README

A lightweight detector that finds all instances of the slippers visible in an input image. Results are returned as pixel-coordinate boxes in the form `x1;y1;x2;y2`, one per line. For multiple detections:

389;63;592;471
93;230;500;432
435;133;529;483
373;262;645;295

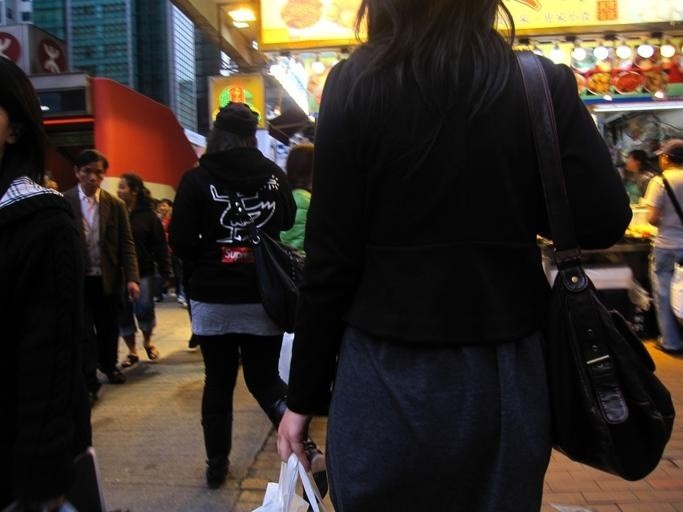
99;343;159;385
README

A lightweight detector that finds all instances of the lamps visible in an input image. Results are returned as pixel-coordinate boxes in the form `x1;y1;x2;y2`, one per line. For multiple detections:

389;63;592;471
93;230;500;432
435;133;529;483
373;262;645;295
593;43;609;60
290;55;304;73
533;45;543;55
311;54;324;73
332;55;341;65
616;40;632;60
570;43;587;62
552;43;564;62
636;40;654;59
270;56;283;75
659;37;676;58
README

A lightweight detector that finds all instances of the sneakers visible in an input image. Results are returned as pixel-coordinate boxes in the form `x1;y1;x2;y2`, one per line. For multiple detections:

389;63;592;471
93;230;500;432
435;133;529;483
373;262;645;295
187;334;200;352
204;461;228;488
301;439;327;503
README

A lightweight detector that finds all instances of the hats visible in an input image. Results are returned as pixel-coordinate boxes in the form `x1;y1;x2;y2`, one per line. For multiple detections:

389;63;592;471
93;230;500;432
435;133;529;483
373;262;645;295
654;138;683;161
215;101;258;137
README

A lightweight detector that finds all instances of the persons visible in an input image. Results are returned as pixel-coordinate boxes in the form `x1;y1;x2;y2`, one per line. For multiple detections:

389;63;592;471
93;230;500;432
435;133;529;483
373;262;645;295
643;139;683;354
168;101;328;512
60;150;141;391
0;56;105;512
118;171;198;367
621;150;654;196
277;0;632;512
280;142;314;255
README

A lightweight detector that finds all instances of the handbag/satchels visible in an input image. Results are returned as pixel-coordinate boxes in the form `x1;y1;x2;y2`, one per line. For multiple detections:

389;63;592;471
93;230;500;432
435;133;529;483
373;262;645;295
252;232;305;334
670;255;683;320
543;259;674;482
